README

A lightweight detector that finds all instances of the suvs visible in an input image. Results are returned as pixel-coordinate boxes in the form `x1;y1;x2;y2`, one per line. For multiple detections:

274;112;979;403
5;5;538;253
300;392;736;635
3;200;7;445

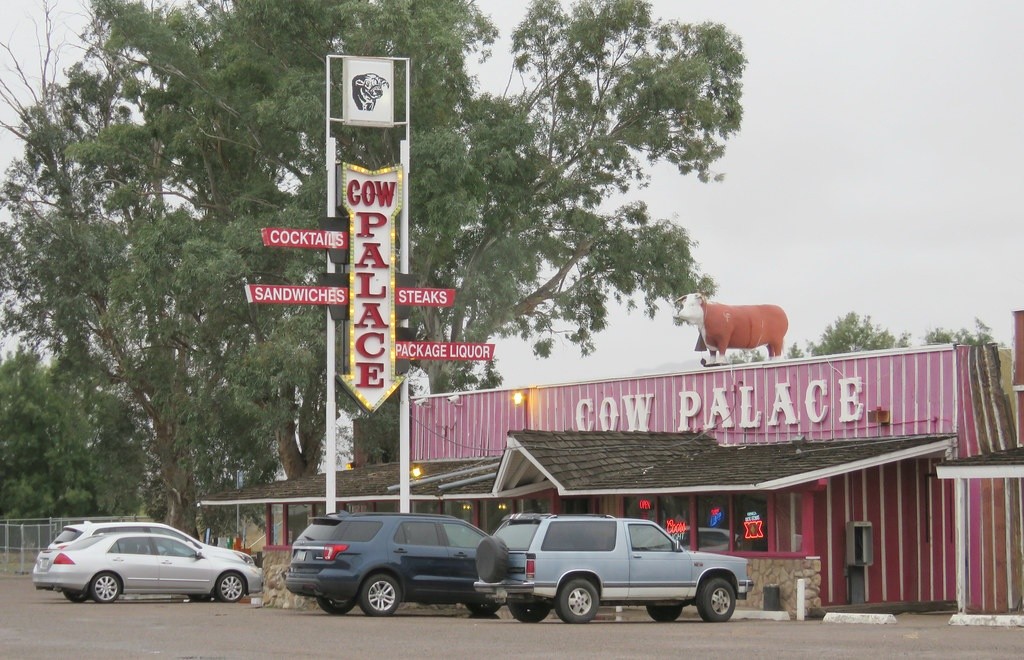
474;513;754;624
645;526;743;553
285;513;503;617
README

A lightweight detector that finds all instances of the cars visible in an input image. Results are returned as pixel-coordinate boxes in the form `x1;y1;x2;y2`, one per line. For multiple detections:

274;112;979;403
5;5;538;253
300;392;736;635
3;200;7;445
32;520;264;603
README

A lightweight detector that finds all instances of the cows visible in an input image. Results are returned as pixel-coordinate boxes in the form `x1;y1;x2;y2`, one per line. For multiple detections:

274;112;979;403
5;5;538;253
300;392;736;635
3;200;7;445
670;293;791;365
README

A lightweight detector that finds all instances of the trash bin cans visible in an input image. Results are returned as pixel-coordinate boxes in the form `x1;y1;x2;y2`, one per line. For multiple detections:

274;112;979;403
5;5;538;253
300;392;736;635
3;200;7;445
763;584;780;611
